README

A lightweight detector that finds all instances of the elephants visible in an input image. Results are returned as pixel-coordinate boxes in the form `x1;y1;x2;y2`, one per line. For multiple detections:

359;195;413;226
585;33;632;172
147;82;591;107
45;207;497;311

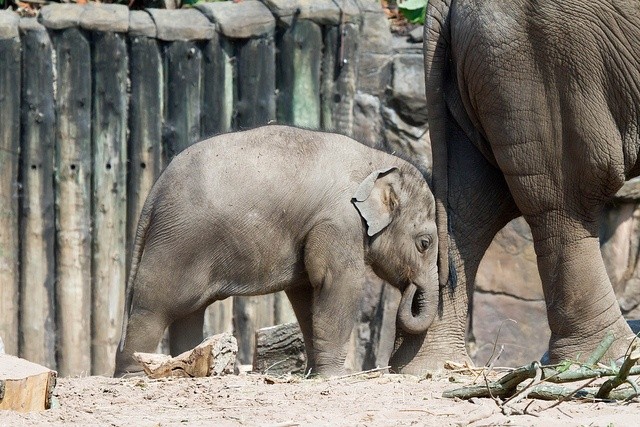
114;122;438;378
387;1;640;374
